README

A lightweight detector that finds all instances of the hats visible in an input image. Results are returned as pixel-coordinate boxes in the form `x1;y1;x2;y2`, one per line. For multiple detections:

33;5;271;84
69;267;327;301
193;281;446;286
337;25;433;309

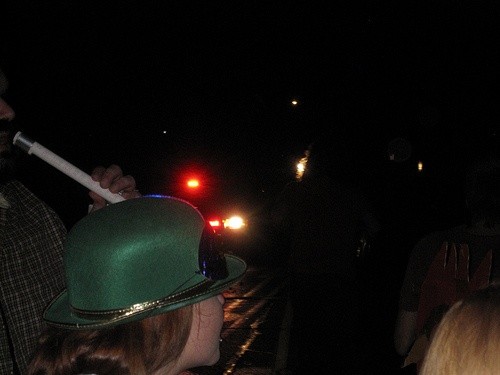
41;195;247;331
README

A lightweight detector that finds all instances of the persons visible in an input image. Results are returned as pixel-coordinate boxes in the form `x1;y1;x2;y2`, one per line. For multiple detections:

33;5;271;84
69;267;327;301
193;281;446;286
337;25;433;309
390;151;500;371
26;194;248;375
1;74;144;374
269;134;382;370
